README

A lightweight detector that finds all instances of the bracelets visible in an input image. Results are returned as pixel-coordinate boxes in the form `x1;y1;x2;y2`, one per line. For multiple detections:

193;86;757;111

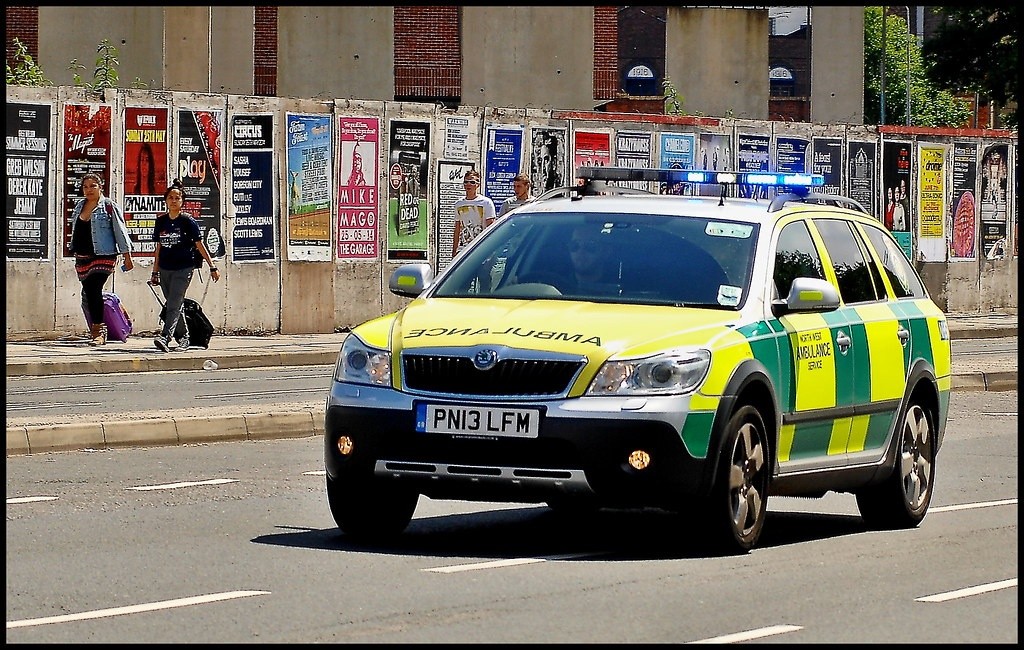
151;272;158;278
211;267;218;272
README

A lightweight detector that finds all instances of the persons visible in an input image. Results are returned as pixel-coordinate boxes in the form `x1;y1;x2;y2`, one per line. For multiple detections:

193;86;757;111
508;223;617;290
133;144;157;196
498;172;536;216
539;136;563;198
407;166;420;196
149;187;221;353
900;179;910;232
347;141;367;186
452;170;495;294
70;174;134;346
892;186;906;231
884;187;895;232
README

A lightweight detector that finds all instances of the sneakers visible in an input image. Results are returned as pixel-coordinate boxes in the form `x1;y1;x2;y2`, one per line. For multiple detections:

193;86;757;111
153;338;171;353
175;339;190;352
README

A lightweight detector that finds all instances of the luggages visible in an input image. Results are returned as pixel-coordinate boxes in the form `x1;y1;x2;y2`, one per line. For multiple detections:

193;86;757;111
146;280;215;350
80;290;133;344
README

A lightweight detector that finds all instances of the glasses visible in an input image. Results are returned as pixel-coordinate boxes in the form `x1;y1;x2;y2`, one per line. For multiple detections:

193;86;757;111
568;240;600;253
464;180;480;186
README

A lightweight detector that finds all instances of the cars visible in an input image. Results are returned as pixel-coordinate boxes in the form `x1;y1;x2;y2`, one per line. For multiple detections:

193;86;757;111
323;168;954;551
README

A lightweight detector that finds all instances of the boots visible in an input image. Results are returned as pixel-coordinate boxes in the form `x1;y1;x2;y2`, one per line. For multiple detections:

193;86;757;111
88;326;95;345
90;324;107;346
